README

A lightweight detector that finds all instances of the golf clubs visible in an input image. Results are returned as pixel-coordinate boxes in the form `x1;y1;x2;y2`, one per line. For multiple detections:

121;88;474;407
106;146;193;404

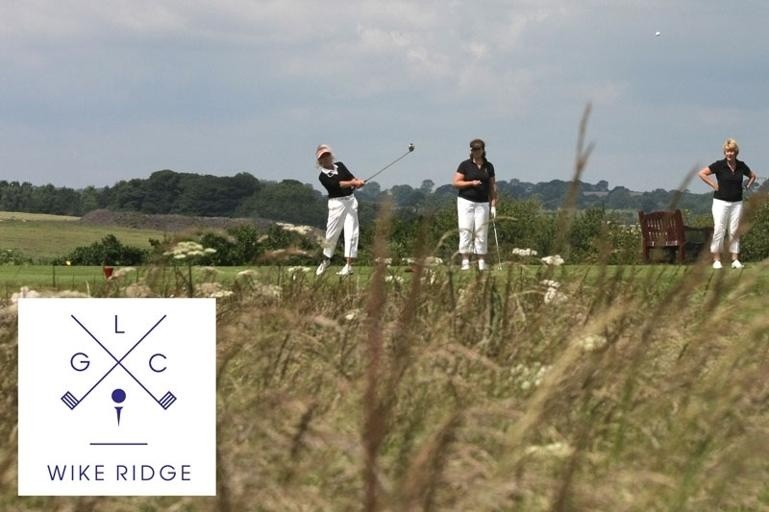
491;214;503;271
352;143;415;189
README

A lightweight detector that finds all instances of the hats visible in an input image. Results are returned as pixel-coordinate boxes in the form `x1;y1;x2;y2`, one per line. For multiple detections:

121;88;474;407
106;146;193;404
469;138;486;148
316;144;331;160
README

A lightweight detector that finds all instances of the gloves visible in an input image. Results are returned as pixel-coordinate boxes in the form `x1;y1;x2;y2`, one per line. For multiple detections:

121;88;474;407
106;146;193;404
490;206;497;219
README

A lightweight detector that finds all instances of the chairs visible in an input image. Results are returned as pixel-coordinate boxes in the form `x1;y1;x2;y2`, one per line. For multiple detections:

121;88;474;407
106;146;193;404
638;208;715;264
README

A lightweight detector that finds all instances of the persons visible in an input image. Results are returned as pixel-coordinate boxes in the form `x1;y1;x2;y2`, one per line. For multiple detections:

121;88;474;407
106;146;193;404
315;144;367;277
451;139;497;271
697;138;756;270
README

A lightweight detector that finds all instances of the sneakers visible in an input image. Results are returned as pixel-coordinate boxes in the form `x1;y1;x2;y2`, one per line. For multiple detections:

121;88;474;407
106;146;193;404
336;263;354;275
712;259;746;270
315;258;334;275
460;259;489;271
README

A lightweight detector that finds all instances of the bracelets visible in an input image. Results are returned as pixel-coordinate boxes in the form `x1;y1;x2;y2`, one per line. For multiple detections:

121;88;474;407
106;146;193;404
744;184;750;191
